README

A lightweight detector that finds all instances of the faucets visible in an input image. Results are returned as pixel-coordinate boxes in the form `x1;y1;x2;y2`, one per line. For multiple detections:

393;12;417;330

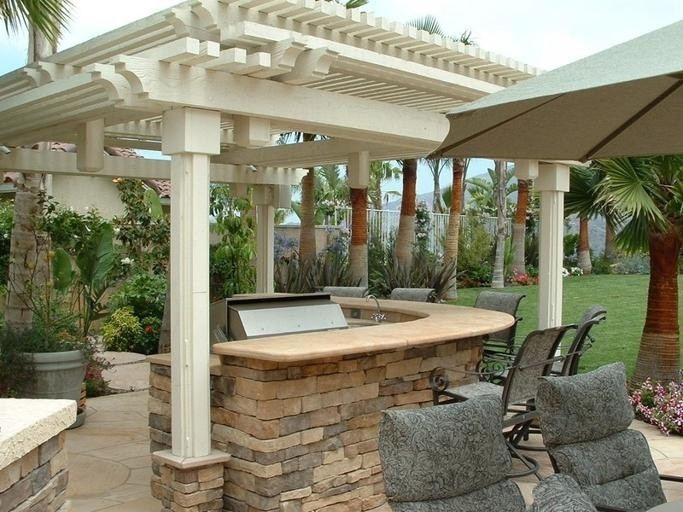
365;294;386;323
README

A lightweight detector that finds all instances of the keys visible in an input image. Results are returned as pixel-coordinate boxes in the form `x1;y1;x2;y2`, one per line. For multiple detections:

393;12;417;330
424;16;683;164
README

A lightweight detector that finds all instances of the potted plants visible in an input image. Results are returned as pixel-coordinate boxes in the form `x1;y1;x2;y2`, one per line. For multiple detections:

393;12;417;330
9;190;85;407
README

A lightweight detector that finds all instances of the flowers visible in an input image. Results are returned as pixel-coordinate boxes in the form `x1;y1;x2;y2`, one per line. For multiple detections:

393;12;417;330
629;376;683;437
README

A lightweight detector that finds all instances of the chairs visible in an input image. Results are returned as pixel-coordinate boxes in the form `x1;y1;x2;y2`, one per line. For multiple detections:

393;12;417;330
389;287;436;303
473;290;527;360
428;303;608;479
378;394;529;511
529;361;681;511
323;285;369;297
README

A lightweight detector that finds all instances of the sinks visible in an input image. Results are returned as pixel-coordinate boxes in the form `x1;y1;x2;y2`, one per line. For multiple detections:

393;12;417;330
339;321;380;329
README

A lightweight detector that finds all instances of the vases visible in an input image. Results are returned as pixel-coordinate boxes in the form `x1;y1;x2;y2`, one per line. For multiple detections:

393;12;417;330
67;380;86;428
